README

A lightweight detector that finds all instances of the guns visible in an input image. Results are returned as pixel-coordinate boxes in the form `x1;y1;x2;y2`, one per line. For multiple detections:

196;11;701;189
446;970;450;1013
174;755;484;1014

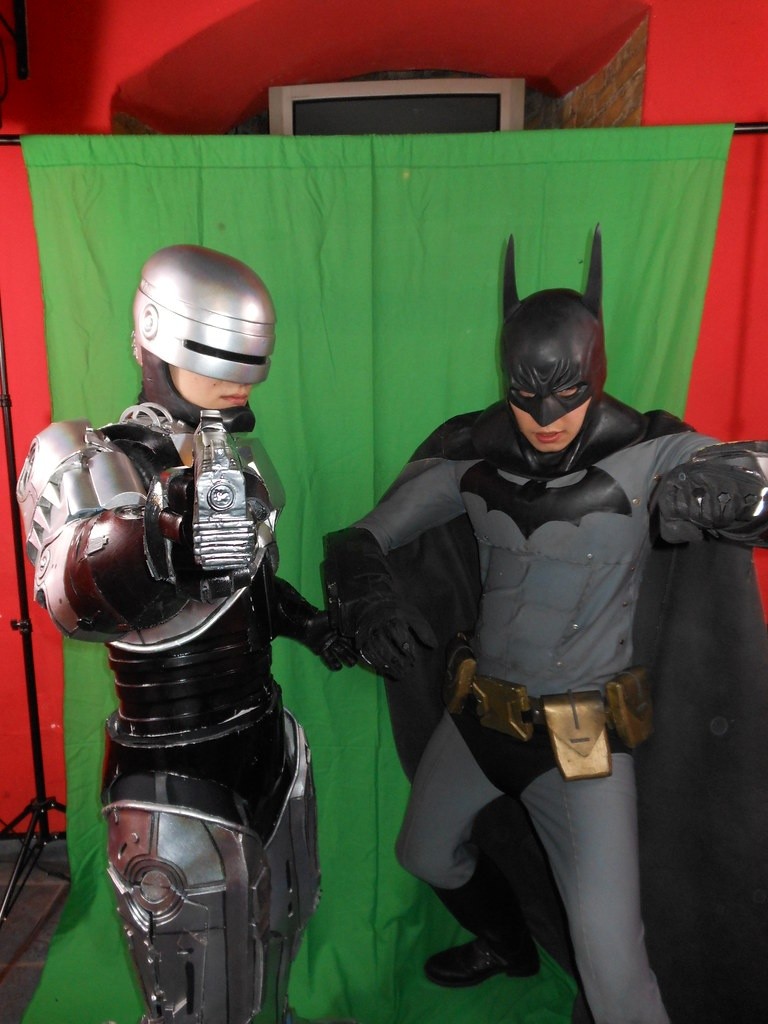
187;408;257;571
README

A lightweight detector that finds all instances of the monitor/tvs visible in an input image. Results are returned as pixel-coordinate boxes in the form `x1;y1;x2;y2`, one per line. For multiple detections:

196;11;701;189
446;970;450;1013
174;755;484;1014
270;79;528;135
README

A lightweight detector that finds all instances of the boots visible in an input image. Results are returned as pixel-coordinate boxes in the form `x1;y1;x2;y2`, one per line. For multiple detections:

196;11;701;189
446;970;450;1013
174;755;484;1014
424;846;543;987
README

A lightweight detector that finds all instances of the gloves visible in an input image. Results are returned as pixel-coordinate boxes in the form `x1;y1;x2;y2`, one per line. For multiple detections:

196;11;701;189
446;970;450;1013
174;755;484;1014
654;462;768;543
322;551;438;679
144;466;280;599
303;612;359;671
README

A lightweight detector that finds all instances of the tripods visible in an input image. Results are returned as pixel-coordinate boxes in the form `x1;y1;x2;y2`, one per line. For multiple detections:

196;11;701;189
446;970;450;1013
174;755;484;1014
0;318;65;930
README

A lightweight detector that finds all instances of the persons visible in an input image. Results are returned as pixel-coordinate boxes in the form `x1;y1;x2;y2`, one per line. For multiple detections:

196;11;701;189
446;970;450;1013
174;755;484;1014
16;245;359;1023
318;230;768;1024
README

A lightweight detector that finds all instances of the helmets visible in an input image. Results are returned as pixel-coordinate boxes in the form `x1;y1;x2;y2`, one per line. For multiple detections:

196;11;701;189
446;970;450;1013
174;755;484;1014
132;244;277;384
